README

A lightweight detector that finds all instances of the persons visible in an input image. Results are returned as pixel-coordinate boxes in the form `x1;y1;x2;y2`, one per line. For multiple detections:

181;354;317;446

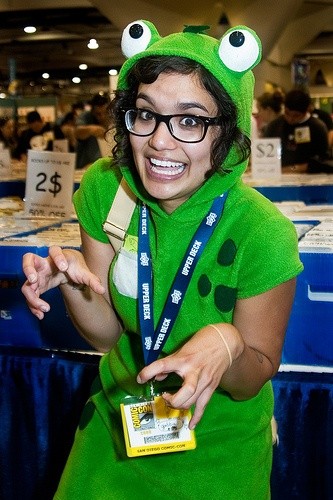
1;86;332;172
20;15;303;500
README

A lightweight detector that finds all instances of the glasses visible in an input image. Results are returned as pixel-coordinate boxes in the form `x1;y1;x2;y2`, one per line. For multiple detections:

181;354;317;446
123;108;222;143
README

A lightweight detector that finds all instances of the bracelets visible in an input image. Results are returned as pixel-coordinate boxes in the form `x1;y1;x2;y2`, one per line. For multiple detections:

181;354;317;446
204;322;234;370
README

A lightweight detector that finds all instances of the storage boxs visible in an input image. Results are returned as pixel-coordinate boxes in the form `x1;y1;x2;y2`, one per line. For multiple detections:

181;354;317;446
0;176;95;352
252;185;333;369
